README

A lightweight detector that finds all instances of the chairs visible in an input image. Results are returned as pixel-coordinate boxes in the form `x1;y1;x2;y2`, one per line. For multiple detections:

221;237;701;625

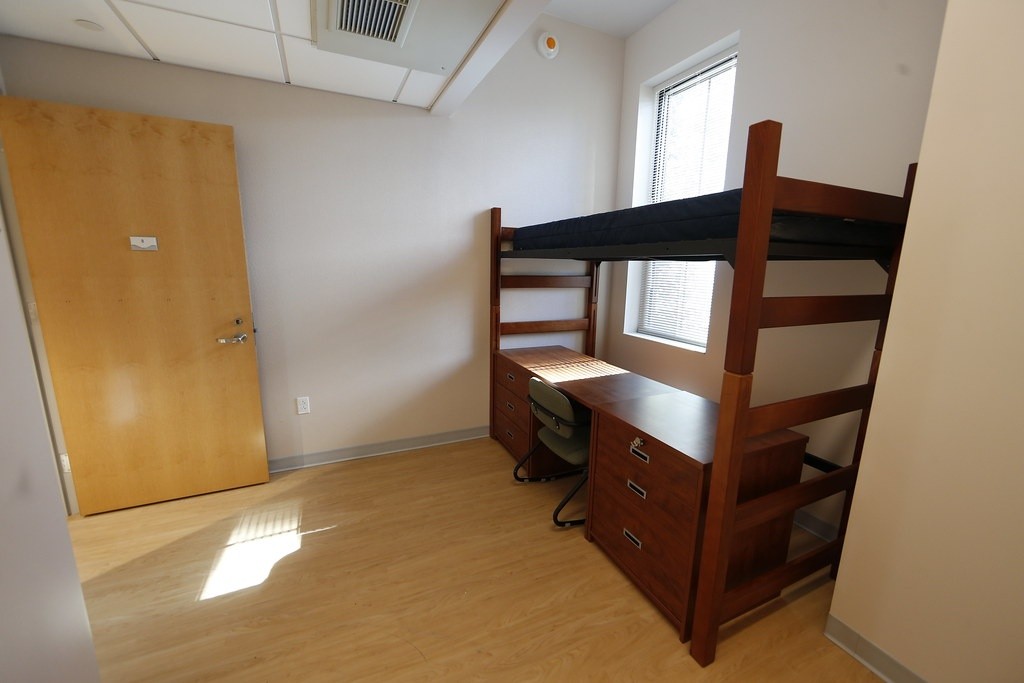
513;377;588;527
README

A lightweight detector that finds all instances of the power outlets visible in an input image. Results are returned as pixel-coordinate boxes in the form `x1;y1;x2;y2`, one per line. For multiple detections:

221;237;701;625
297;396;310;415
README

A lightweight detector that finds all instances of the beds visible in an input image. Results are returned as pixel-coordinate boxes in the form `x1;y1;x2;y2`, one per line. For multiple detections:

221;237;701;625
489;122;917;668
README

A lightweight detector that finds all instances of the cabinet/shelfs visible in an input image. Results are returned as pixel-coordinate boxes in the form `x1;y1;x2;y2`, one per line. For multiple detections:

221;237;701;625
491;346;809;645
